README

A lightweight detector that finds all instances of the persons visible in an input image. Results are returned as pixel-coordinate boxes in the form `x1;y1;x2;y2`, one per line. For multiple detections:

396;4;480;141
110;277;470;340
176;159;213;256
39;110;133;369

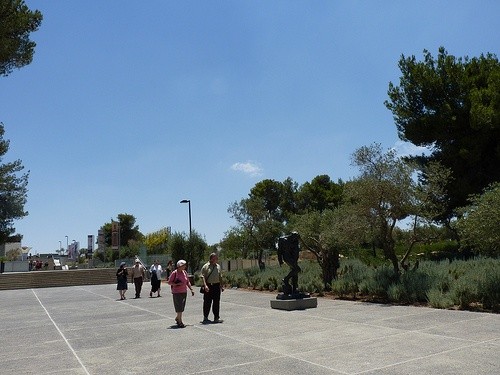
30;256;49;270
278;230;303;293
149;259;166;297
167;260;194;328
200;253;225;324
166;261;172;280
131;259;145;299
116;262;129;300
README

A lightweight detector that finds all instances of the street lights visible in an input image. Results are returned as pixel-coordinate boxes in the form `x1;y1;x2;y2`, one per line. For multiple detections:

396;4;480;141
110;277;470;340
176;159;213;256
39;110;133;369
179;200;192;243
58;240;62;250
64;235;68;246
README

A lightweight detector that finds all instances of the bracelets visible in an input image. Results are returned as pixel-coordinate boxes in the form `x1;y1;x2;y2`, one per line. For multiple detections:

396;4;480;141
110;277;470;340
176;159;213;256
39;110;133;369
190;288;193;290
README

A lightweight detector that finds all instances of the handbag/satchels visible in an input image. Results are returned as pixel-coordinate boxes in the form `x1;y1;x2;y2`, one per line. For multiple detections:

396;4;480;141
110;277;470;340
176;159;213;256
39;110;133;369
200;285;206;293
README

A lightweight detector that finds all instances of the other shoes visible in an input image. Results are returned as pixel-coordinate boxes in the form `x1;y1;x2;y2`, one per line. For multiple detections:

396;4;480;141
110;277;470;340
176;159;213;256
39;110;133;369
175;318;185;328
214;319;223;323
203;319;214;323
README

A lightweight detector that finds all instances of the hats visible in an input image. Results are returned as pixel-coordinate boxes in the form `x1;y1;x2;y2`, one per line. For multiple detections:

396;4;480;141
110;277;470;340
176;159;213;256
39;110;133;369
135;259;140;263
177;260;187;267
